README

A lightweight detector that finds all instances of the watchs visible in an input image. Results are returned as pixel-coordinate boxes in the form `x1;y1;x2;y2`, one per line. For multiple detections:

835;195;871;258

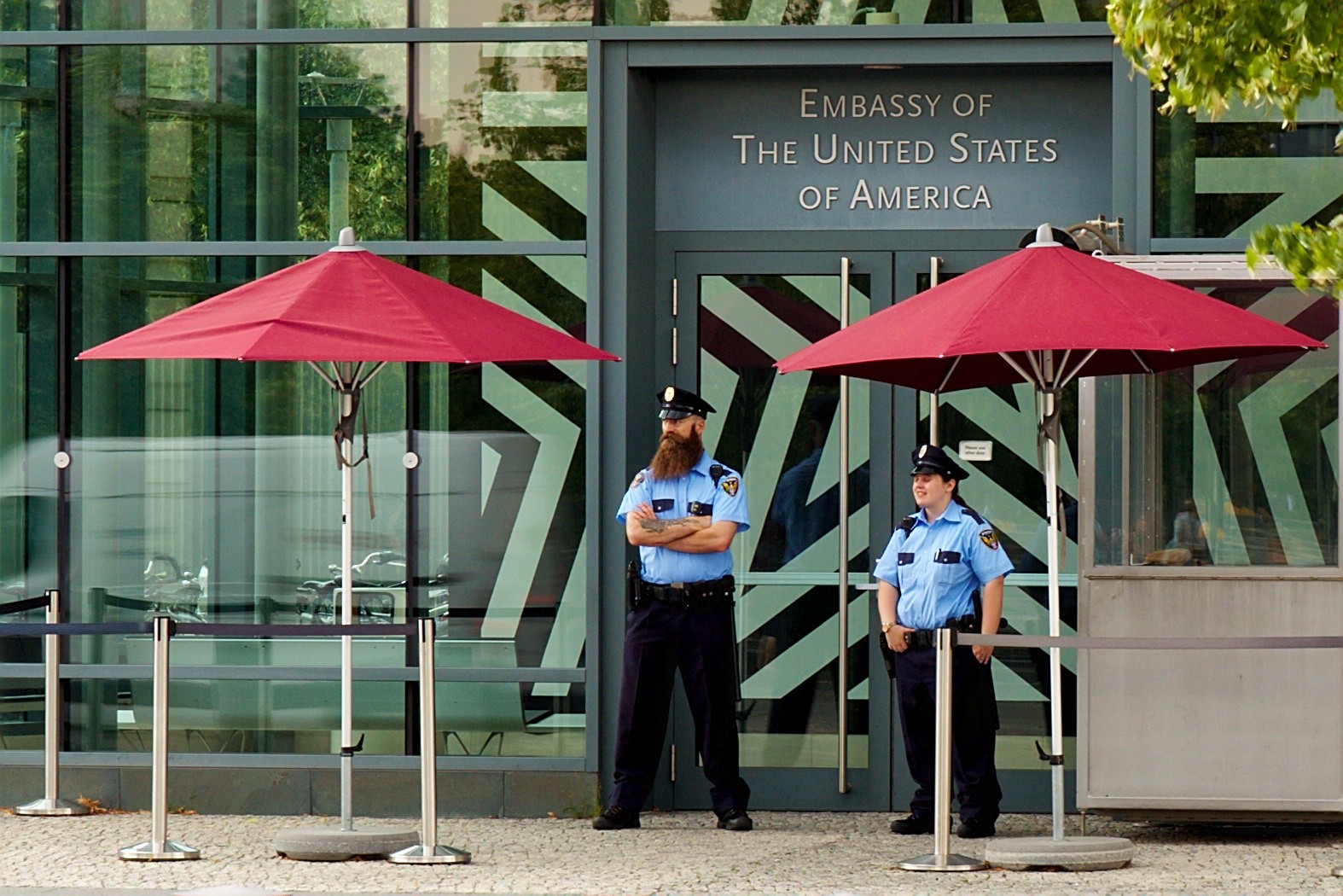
882;622;897;633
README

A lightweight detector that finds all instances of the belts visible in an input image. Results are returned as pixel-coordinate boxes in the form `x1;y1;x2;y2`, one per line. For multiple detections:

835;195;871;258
903;621;982;648
640;582;733;606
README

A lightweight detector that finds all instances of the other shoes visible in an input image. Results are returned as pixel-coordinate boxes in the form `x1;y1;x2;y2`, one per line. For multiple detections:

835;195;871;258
890;813;954;835
957;821;996;839
717;812;753;831
592;811;641;831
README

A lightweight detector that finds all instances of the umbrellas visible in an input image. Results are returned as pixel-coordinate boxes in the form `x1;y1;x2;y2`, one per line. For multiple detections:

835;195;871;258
72;224;623;863
771;221;1330;873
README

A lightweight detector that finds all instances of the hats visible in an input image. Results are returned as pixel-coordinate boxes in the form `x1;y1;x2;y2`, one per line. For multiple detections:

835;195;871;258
909;444;970;482
656;385;716;420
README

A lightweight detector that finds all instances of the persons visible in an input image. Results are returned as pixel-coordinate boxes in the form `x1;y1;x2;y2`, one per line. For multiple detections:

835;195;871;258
872;445;1016;838
590;385;755;833
